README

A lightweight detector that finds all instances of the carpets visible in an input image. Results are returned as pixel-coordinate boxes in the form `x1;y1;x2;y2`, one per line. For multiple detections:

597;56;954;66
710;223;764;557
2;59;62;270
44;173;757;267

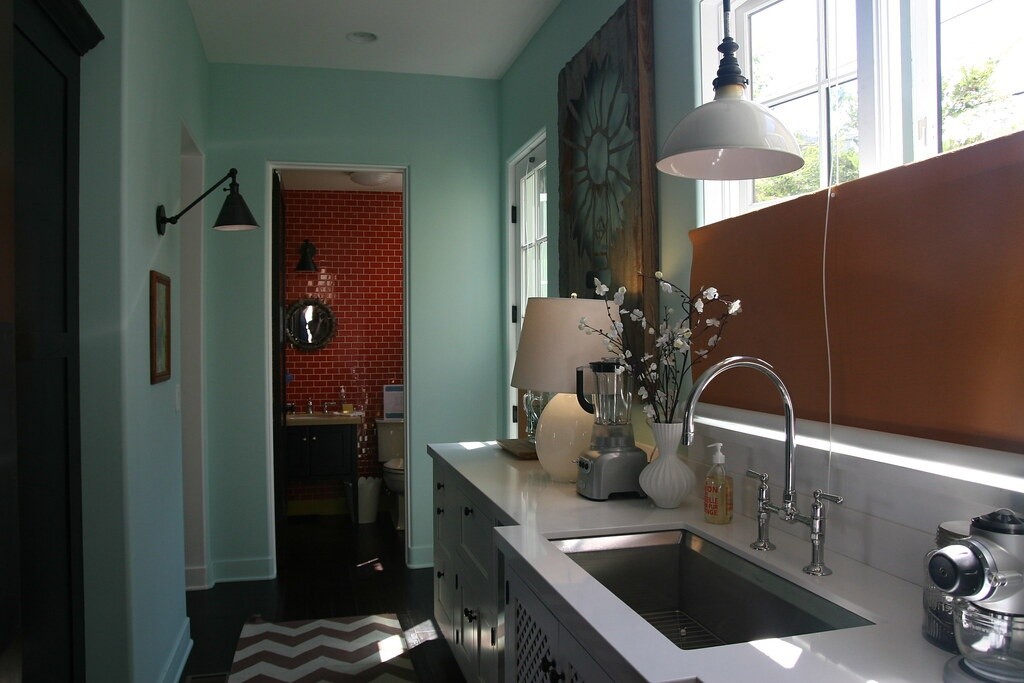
226;613;420;683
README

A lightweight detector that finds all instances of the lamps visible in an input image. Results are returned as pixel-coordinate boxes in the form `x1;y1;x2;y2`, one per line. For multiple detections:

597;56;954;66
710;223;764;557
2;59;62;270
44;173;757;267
295;240;318;271
351;171;392;186
511;298;625;484
655;0;805;180
155;168;262;235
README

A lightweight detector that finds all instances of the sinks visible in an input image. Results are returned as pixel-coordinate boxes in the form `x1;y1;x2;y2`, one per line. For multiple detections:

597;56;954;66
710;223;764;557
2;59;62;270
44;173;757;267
549;528;877;651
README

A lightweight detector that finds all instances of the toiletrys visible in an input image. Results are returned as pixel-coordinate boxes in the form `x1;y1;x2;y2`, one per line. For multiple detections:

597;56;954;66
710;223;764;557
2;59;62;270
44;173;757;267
338;385;347;412
704;442;734;525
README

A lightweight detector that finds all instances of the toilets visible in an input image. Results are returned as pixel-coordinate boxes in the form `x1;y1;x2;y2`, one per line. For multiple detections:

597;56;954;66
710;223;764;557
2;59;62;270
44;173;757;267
375;419;405;530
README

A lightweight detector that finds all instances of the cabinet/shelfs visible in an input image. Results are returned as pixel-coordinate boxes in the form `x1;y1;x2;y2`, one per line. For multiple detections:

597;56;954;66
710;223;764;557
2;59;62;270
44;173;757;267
286;425;356;477
427;442;498;683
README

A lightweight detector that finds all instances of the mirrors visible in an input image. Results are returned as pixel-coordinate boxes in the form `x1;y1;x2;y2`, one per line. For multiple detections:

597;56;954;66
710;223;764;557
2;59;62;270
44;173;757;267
284;299;335;348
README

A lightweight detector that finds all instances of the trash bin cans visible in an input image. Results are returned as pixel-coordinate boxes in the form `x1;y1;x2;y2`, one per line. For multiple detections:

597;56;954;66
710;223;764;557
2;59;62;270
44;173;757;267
345;459;384;529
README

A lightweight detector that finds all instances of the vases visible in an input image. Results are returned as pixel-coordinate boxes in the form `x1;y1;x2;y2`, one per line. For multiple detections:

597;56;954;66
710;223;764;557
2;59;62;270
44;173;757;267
639;421;702;508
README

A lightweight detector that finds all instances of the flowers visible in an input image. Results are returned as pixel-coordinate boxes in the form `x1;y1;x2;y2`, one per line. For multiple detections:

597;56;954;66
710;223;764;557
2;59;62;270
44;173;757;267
579;271;745;424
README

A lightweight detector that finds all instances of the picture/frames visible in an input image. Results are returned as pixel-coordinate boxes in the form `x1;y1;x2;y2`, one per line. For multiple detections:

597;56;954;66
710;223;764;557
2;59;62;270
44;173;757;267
149;270;173;385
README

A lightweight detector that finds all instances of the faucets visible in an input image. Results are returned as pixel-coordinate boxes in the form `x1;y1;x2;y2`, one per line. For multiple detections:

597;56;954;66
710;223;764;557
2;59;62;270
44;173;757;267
306;399;314;414
681;355;845;577
